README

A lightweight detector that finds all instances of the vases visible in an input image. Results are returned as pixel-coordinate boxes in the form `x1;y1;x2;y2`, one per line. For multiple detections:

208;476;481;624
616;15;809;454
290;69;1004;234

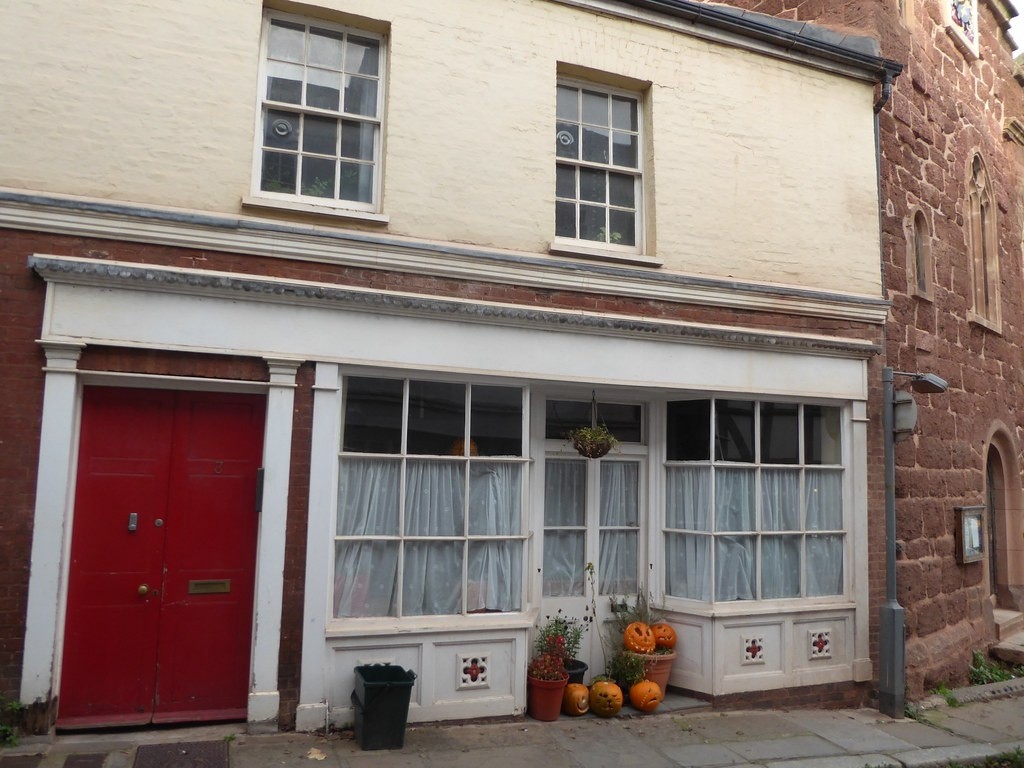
352;664;417;752
526;673;569;721
563;656;588;684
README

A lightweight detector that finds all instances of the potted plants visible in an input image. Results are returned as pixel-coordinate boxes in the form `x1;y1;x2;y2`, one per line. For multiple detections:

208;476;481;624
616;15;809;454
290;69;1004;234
568;426;624;459
598;583;679;702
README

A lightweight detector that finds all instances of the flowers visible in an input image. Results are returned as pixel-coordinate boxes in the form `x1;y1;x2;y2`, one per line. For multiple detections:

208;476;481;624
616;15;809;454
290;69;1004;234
527;605;593;680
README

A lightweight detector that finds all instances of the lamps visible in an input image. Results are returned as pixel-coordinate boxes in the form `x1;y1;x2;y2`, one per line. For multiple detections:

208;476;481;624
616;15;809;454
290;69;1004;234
894;371;949;393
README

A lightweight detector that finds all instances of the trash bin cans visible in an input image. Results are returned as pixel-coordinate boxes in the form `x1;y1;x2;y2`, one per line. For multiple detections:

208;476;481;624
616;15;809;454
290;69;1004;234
351;664;418;751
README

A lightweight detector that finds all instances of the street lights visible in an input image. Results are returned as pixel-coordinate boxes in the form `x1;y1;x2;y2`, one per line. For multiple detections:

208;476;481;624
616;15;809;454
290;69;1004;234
879;365;951;720
